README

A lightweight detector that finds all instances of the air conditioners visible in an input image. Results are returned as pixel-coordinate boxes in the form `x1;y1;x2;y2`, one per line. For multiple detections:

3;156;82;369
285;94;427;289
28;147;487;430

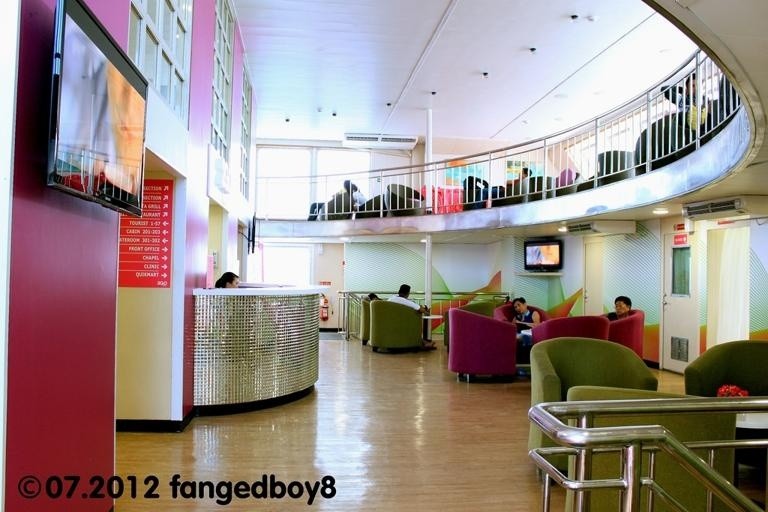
680;195;768;219
568;220;637;237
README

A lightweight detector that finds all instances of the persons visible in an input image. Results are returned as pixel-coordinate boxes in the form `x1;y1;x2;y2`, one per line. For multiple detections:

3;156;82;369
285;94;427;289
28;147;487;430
510;297;541;377
385;283;437;350
61;60;146;212
604;295;632;322
658;71;712;145
462;174;488;190
469;167;532;208
213;271;240;288
306;179;357;221
368;293;379;301
532;244;560;266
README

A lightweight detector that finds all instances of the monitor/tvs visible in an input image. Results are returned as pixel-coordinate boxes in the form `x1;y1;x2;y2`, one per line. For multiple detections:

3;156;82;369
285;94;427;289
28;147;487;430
43;1;149;220
524;239;563;273
252;216;255;253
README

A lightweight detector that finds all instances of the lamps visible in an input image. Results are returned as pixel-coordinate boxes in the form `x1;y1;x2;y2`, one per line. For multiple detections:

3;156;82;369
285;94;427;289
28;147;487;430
558;220;568;233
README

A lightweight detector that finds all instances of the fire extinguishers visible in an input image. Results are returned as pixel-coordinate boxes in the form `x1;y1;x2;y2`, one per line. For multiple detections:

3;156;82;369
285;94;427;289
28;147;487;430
320;294;329;321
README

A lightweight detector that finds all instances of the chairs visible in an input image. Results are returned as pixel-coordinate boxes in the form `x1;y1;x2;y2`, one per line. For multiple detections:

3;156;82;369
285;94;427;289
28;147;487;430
357;294;768;512
316;73;742;218
